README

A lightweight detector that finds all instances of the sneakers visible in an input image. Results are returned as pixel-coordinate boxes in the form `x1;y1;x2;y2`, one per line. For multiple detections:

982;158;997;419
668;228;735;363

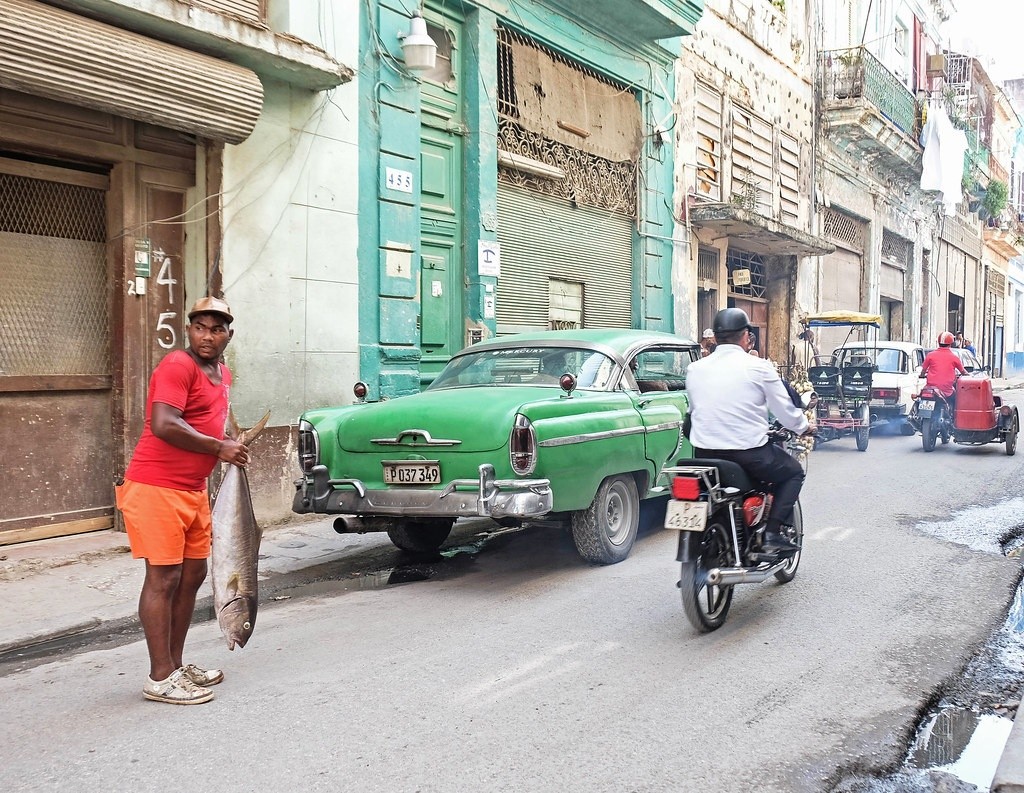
177;663;224;687
142;670;214;705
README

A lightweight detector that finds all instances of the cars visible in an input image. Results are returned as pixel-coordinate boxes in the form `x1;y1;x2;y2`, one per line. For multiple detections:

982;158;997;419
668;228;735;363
290;327;702;565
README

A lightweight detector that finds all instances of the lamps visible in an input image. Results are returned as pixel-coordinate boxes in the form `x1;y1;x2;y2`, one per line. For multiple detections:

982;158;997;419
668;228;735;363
396;9;439;70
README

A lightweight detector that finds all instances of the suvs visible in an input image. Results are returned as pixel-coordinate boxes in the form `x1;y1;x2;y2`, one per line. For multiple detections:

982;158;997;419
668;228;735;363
925;348;991;378
829;340;929;436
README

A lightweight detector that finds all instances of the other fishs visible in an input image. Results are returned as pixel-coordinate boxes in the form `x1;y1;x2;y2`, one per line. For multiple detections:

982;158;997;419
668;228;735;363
209;400;272;652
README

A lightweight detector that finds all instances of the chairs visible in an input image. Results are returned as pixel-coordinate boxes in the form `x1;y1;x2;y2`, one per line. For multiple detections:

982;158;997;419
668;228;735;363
841;355;873;398
636;381;669;393
852;354;868;365
808;354;840;395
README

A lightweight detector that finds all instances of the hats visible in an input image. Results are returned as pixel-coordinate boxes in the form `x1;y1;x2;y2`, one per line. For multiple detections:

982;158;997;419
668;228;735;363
703;328;714;338
964;337;972;344
188;296;234;325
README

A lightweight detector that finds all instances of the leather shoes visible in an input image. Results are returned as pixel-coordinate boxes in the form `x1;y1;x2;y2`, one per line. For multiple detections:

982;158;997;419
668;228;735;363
765;531;802;550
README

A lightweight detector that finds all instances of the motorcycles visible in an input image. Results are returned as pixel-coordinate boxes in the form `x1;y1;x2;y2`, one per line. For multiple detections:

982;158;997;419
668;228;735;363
797;310;885;451
658;378;818;633
907;365;1020;456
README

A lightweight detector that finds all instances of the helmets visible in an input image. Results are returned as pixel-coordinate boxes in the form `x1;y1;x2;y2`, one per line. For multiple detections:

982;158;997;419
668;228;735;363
938;331;955;344
713;308;753;332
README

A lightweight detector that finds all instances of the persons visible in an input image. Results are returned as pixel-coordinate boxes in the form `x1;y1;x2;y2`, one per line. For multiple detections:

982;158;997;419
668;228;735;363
115;297;251;707
686;305;820;553
920;330;976;423
